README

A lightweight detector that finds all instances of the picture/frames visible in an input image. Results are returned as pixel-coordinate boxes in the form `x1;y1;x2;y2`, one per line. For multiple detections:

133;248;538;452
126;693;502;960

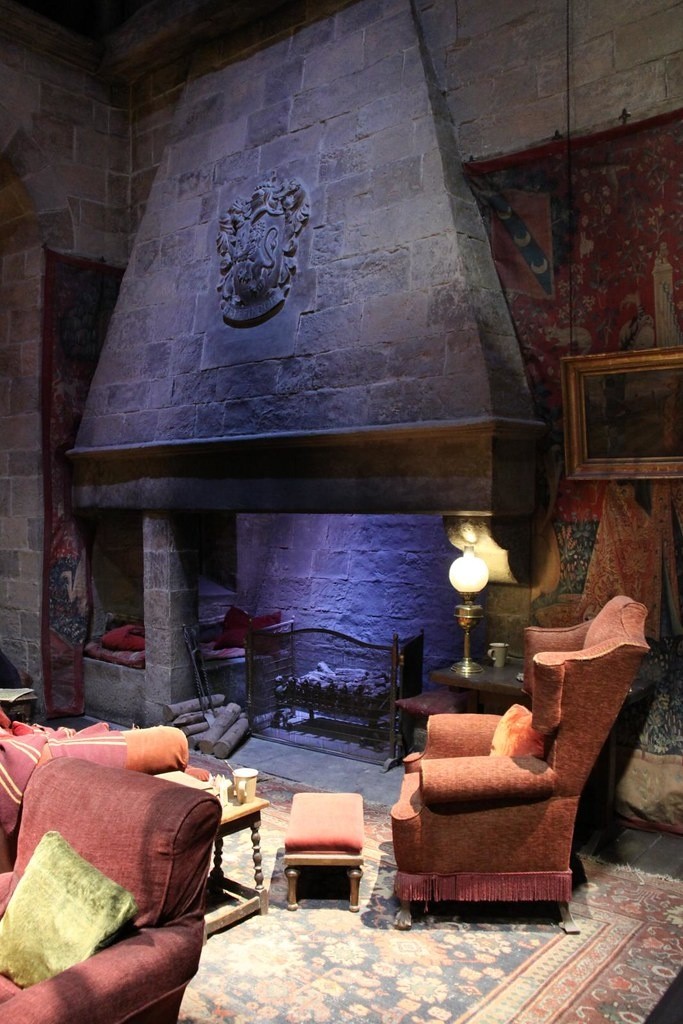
560;344;683;481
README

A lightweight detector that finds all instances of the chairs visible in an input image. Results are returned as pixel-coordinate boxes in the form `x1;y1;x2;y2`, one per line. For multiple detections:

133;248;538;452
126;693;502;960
388;595;650;934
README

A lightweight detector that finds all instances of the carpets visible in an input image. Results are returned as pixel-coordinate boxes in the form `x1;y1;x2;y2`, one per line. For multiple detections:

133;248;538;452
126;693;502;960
177;751;683;1024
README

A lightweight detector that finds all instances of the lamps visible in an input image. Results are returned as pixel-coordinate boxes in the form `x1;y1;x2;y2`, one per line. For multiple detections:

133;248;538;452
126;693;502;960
449;546;489;674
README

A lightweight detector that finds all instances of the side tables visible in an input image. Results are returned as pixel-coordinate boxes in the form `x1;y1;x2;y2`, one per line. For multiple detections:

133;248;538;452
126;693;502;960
430;664;531;716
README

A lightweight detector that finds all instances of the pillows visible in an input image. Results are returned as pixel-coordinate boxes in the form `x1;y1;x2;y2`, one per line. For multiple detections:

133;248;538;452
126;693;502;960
100;624;144;650
488;703;544;759
211;606;281;658
0;831;138;988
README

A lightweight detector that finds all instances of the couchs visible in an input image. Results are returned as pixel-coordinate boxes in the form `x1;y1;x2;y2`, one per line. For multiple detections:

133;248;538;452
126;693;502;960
0;756;223;1024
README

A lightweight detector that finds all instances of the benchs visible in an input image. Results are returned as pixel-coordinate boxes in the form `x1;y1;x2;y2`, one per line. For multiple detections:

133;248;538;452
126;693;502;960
0;705;210;874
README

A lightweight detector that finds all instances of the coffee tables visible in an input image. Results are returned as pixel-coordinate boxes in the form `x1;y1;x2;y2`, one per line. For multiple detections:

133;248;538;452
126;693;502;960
153;771;270;947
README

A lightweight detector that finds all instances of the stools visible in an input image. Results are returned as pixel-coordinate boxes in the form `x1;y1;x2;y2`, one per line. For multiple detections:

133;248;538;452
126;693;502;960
283;792;364;912
